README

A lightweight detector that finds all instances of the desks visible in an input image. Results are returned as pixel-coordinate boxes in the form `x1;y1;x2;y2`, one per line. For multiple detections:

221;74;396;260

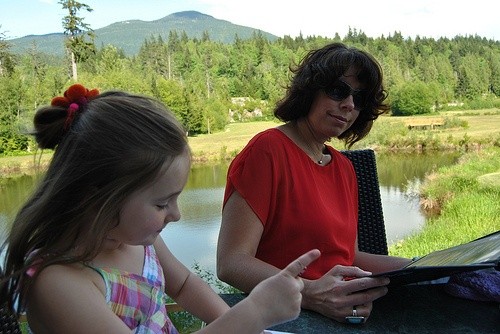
214;293;499;334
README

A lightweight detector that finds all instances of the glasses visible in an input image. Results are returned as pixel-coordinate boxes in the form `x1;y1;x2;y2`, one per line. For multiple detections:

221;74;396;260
312;80;372;110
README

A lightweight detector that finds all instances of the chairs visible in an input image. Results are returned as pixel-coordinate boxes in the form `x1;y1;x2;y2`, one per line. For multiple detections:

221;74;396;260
340;149;388;255
0;268;185;334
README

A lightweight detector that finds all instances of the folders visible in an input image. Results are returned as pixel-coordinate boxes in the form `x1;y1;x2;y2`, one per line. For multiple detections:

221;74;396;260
369;230;500;285
